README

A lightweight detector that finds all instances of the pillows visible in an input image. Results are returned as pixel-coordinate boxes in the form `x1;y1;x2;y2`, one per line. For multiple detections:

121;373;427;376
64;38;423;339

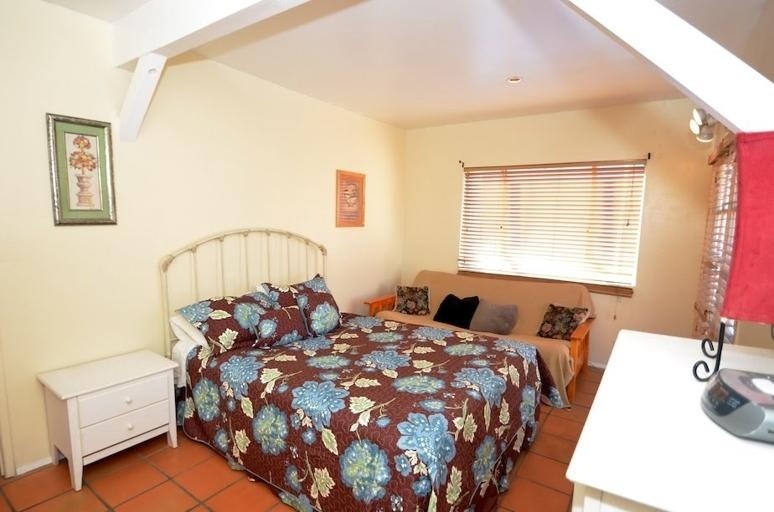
171;324;190;341
536;302;591;340
433;294;480;330
393;285;430;315
469;297;518;335
248;304;309;351
174;290;280;357
170;316;209;349
287;273;343;337
259;279;298;307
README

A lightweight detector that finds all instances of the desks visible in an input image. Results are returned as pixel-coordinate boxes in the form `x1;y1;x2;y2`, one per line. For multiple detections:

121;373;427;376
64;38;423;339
565;329;773;512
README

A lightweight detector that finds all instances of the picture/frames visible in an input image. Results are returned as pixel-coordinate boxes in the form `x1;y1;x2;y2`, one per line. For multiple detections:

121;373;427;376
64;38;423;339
47;113;117;224
336;169;365;228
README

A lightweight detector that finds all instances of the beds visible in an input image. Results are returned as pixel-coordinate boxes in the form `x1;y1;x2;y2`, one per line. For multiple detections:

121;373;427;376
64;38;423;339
158;228;570;512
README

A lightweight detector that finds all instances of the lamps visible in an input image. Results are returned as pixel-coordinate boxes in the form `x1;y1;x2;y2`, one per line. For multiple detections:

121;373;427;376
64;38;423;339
689;107;719;143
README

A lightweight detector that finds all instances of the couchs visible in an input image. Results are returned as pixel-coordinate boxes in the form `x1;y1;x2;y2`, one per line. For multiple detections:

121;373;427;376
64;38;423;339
364;269;596;405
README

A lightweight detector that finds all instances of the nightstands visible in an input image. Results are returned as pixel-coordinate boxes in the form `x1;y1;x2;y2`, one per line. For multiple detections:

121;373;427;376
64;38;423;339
36;349;179;492
